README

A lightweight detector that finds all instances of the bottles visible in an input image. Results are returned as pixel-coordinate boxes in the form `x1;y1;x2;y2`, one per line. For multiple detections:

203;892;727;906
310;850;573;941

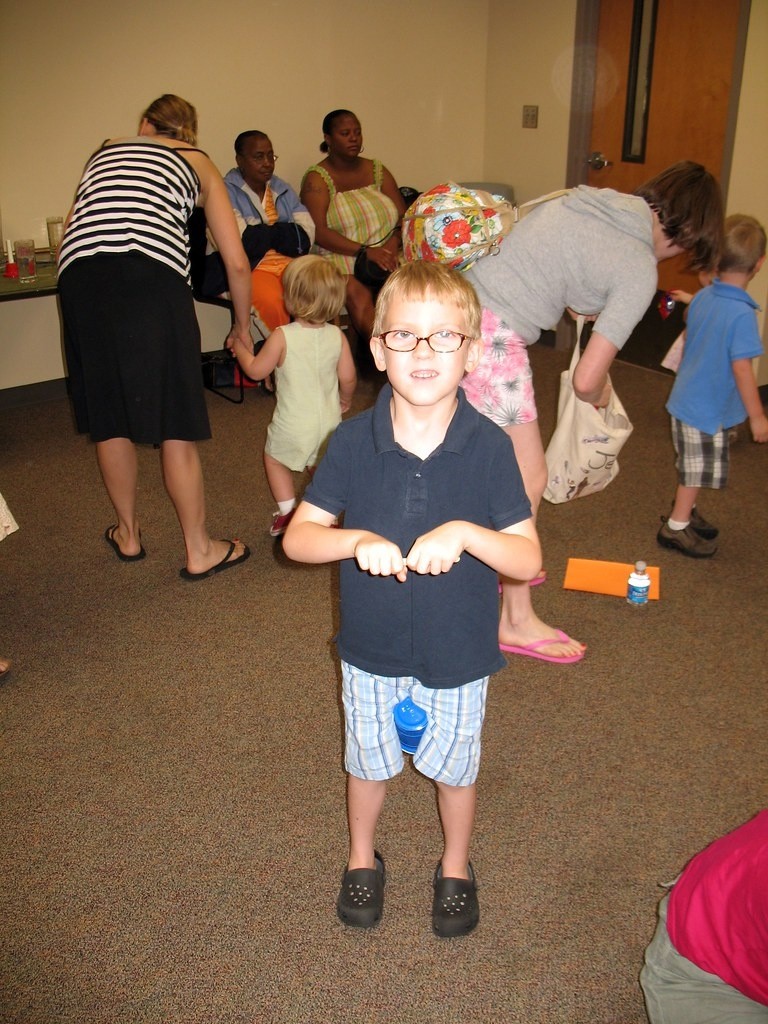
625;560;650;616
394;696;427;753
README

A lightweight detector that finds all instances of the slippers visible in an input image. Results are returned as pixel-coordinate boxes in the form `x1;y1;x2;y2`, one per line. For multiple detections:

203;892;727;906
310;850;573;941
497;628;587;663
498;568;546;593
180;537;250;581
105;523;145;561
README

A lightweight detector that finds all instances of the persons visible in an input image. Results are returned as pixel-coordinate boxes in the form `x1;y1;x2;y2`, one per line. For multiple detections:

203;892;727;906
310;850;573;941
231;254;357;536
300;109;408;378
0;492;20;678
283;258;542;936
456;161;720;663
638;809;768;1024
661;213;768;557
207;130;315;395
54;95;254;582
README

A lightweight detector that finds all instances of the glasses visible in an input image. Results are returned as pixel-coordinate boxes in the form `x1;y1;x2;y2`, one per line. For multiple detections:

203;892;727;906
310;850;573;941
238;153;278;162
378;330;473;353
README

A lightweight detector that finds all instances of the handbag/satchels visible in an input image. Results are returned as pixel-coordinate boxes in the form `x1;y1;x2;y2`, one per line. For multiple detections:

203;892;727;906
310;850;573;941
354;227;402;287
199;342;261;403
542;314;633;504
396;176;571;274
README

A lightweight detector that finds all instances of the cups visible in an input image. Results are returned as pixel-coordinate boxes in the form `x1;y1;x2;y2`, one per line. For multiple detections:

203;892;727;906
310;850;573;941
45;216;63;255
14;240;38;284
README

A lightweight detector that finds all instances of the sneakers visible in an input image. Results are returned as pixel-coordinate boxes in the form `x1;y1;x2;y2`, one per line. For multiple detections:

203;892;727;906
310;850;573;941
670;499;718;538
658;516;717;559
270;504;299;537
329;522;340;528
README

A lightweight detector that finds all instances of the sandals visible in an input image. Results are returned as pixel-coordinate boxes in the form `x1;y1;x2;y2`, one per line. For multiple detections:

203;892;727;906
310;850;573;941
259;373;275;395
337;849;385;926
0;661;11;686
433;856;479;936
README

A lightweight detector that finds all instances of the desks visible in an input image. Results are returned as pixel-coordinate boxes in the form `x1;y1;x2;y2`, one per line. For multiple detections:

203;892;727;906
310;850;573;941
0;257;69;390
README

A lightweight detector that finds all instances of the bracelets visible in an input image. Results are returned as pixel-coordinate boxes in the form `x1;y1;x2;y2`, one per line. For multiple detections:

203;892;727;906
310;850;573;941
356;243;367;258
394;226;401;231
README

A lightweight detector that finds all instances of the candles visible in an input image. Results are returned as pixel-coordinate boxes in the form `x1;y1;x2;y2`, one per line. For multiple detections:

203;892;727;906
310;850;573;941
7;239;14;264
